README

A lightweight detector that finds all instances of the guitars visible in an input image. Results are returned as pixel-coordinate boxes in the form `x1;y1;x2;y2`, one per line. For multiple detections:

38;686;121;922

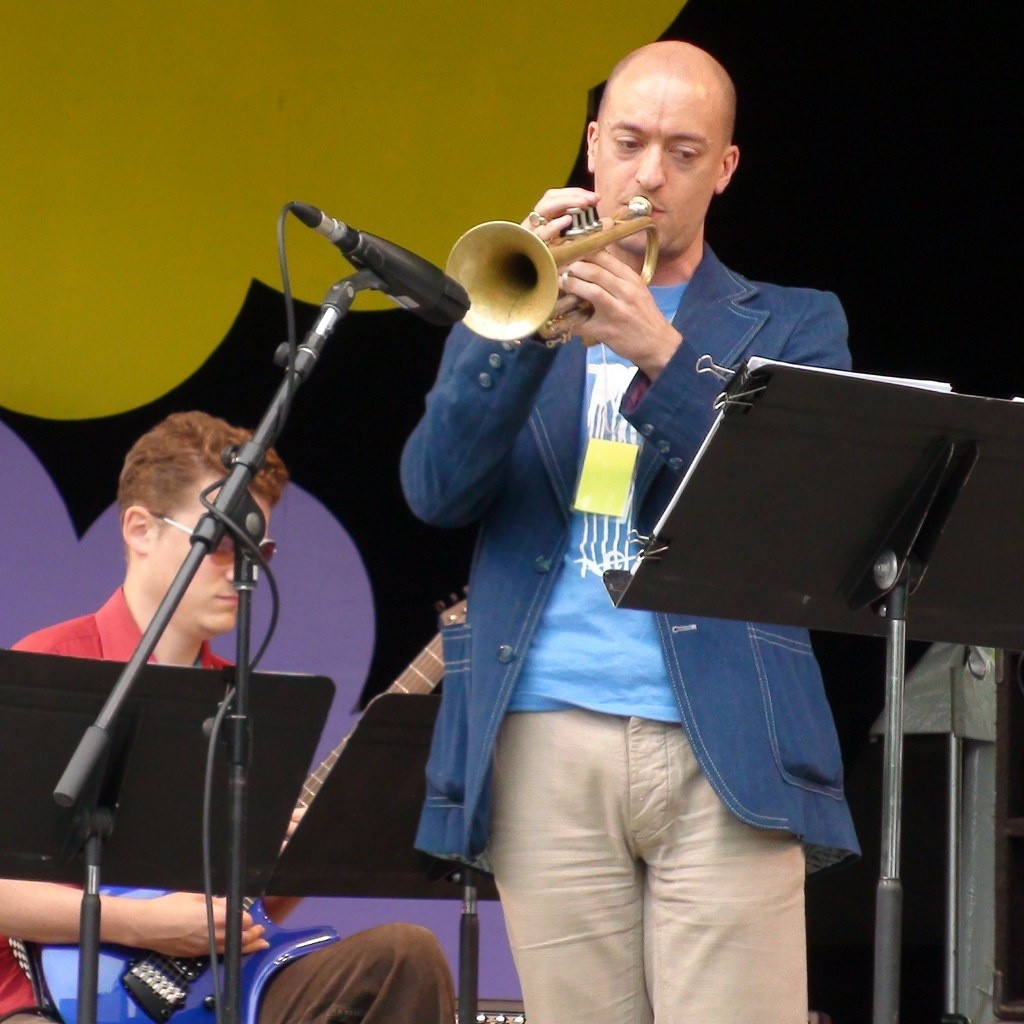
29;589;484;1023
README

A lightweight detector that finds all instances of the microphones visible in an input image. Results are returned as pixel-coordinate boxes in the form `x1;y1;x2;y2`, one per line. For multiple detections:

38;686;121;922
291;202;471;327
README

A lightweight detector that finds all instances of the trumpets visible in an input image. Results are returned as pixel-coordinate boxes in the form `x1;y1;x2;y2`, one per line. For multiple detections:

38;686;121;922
445;186;667;355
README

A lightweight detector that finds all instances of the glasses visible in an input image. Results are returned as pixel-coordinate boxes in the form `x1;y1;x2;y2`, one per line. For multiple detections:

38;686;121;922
146;510;278;568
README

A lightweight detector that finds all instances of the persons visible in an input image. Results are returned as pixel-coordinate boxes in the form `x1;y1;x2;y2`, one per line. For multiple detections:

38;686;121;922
0;413;457;1024
401;40;860;1024
